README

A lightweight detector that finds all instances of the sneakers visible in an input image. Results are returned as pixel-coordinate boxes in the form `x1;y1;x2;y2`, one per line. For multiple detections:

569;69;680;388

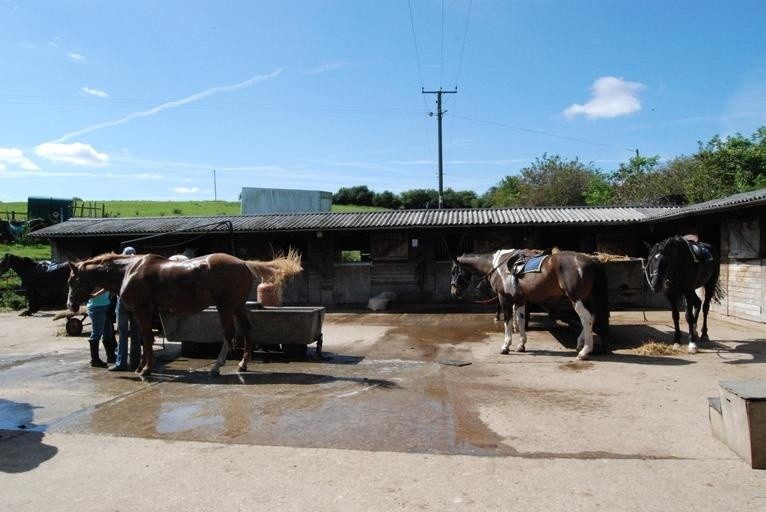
108;365;127;371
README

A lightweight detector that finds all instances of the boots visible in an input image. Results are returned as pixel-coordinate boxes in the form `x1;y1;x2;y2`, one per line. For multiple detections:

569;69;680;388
90;341;107;367
103;342;116;363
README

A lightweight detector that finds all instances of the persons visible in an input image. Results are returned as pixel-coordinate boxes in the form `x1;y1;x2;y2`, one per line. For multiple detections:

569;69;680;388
87;284;118;368
108;246;141;371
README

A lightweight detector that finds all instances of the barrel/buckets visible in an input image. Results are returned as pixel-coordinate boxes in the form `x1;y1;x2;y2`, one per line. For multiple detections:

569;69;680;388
255;277;282;306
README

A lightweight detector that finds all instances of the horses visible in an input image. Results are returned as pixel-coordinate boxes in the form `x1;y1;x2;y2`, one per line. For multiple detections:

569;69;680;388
0;252;68;317
448;247;610;360
642;235;726;355
63;244;305;378
